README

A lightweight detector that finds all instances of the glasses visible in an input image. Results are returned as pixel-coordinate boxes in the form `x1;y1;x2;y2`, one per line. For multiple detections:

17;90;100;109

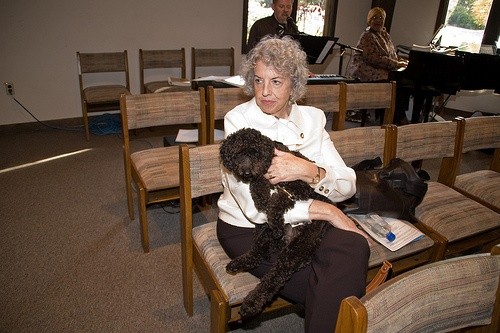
371;16;384;20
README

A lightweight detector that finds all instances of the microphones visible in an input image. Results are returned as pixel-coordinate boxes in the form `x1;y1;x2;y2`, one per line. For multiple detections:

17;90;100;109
287;17;298;29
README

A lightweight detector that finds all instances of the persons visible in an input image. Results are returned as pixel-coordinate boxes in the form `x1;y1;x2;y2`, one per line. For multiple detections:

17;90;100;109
348;7;409;126
217;34;371;333
248;0;298;48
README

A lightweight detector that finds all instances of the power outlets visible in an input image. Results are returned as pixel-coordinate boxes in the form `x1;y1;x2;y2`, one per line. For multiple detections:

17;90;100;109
5;82;15;95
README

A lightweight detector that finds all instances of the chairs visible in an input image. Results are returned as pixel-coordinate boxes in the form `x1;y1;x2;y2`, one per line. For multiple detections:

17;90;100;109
75;47;500;333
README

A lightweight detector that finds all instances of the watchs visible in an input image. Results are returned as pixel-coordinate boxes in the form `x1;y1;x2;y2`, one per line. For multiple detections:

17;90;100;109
307;165;321;186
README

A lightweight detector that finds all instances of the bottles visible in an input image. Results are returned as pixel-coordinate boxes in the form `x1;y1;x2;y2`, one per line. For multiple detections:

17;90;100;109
365;212;395;241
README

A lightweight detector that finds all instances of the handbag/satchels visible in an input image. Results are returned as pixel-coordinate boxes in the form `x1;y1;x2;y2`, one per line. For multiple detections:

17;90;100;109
336;157;429;224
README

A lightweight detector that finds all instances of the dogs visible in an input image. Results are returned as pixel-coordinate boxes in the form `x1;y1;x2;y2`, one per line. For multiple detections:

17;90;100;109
220;128;335;324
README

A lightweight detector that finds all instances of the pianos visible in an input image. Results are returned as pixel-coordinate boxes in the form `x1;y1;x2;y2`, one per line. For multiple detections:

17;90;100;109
394;42;500;126
